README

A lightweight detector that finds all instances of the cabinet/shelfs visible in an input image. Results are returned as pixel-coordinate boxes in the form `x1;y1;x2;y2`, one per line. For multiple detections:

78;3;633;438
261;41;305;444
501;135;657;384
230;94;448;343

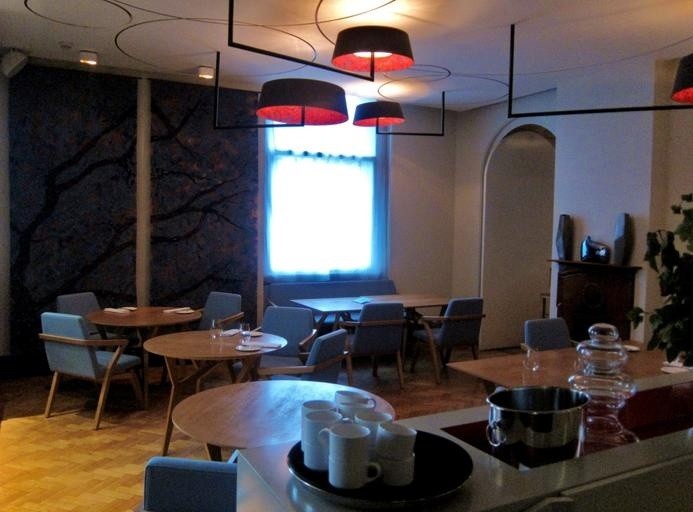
547;259;643;343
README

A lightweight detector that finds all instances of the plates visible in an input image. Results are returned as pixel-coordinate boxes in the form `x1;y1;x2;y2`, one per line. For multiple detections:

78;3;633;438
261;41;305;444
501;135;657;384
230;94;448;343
235;345;262;352
242;330;263;336
285;431;475;505
122;305;136;312
623;344;640;351
660;365;690;374
175;311;196;314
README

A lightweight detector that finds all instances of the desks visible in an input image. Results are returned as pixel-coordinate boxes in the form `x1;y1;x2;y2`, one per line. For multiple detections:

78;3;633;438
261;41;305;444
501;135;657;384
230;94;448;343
86;304;202;411
142;326;288;462
167;378;396;463
447;339;691;390
286;296;455;371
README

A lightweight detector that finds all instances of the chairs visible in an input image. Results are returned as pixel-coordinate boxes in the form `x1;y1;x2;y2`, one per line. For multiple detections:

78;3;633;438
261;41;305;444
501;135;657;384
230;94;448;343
57;290;139;355
41;310;146;430
410;296;485;374
266;278;396;310
259;329;353;390
196;290;245;331
142;447;240;512
337;301;407;390
230;305;316;375
520;317;582;353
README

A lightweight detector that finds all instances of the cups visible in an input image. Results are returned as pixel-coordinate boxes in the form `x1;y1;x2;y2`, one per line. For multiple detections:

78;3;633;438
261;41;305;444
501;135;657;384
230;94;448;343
334;402;376;417
238;322;251;347
379;454;415;486
317;422;371;455
300;399;337;411
303;442;329;471
522;347;541;371
354;409;393;421
335;389;385;410
208;317;223;344
328;455;382;489
303;411;343;441
375;421;416;453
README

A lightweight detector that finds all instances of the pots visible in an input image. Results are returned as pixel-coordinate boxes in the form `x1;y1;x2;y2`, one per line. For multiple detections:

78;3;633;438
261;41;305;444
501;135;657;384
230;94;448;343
487;385;591;469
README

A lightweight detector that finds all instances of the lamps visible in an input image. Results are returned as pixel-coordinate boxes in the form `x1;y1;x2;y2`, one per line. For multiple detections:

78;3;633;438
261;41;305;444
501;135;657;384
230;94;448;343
226;1;415;84
507;25;693;119
353;90;446;136
214;50;349;130
